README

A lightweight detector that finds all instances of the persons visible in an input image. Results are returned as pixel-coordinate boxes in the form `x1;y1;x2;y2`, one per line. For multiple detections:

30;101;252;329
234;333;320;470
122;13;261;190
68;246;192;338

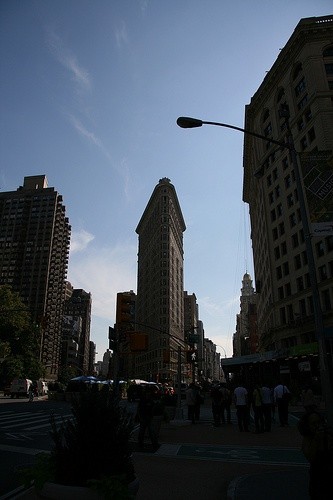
188;383;204;423
301;384;314;412
211;378;291;433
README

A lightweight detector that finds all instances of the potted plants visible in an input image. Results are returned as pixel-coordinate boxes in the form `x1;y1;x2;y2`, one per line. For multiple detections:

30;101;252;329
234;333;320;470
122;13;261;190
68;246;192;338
26;381;141;499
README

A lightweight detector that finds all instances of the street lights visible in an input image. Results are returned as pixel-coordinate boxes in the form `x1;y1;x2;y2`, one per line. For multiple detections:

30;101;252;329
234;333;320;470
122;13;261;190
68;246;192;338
174;115;333;423
119;317;202;422
